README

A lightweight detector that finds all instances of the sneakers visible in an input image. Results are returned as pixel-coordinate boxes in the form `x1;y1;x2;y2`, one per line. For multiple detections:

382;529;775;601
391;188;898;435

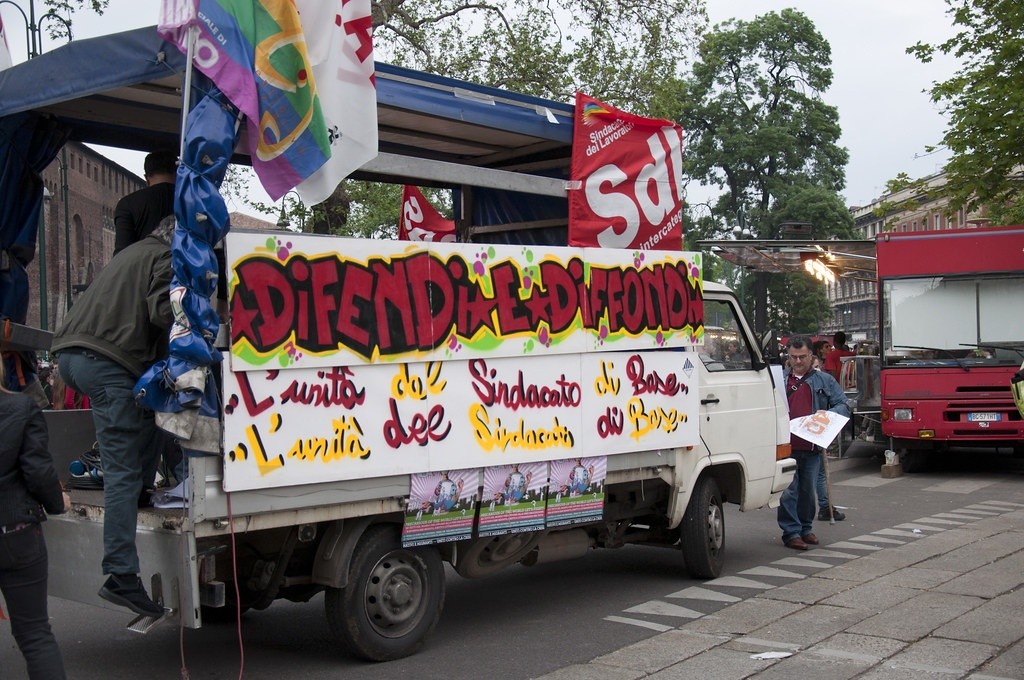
97;575;163;617
137;496;152;509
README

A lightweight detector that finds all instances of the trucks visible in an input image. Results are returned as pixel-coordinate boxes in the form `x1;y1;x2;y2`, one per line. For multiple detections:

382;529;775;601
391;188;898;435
0;25;796;662
695;227;1024;478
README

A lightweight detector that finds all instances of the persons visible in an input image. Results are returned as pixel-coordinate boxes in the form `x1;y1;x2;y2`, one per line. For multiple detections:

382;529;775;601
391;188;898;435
50;213;176;617
36;358;91;409
112;149;178;255
810;356;845;521
812;332;857;377
0;357;70;680
779;336;849;549
697;334;742;370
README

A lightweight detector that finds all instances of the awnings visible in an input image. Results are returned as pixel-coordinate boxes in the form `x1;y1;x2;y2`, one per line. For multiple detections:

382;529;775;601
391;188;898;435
694;240;877;281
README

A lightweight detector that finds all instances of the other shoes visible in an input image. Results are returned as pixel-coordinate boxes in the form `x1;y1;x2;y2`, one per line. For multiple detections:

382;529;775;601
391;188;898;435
818;507;844;521
801;533;819;545
787;537;808;549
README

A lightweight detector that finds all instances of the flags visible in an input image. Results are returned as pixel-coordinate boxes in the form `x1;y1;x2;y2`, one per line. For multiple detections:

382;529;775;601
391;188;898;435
159;1;379;206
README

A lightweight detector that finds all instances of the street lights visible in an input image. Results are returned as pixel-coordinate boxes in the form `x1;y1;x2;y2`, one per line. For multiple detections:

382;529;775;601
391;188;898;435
53;146;71;313
1;1;73;330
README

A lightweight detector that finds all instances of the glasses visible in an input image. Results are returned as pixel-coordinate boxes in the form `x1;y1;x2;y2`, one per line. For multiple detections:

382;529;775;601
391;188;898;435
825;345;832;348
